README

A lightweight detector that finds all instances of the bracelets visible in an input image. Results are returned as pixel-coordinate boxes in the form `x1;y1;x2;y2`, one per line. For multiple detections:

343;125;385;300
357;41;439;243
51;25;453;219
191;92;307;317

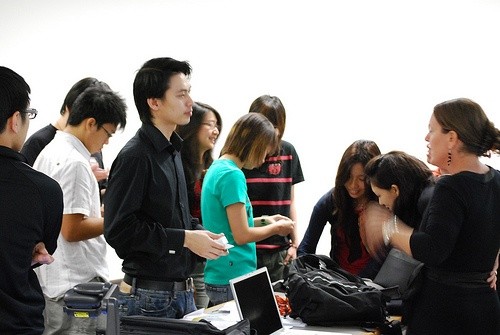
261;215;268;225
381;215;398;248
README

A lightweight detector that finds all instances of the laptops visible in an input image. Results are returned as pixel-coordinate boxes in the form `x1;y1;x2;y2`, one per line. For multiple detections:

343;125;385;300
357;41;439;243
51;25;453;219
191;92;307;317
229;266;353;335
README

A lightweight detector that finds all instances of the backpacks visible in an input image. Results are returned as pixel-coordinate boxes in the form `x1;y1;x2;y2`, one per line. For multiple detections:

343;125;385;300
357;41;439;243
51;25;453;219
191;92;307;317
283;254;401;329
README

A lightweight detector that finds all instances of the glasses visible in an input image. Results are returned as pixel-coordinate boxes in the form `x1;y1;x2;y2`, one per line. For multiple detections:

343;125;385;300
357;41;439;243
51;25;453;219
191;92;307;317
19;108;38;119
203;122;220;130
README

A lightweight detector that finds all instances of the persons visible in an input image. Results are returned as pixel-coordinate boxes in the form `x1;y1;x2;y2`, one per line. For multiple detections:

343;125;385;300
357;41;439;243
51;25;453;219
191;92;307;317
20;76;112;217
104;58;229;320
359;97;500;335
297;140;393;282
240;94;305;283
200;113;295;305
32;87;128;335
0;66;64;335
176;102;223;308
364;151;436;231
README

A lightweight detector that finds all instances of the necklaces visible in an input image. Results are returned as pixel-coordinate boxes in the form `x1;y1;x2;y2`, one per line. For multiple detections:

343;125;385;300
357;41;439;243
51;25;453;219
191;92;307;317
357;200;370;206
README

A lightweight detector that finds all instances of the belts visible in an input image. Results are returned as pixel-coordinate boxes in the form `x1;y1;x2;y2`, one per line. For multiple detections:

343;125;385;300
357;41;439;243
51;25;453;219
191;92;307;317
123;274;193;292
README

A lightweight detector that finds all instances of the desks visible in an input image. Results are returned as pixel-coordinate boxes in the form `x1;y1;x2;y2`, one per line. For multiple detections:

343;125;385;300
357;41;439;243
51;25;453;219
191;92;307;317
179;292;407;335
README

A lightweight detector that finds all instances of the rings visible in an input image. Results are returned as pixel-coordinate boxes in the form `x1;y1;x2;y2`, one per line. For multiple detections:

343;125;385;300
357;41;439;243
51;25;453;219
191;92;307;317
495;271;497;275
